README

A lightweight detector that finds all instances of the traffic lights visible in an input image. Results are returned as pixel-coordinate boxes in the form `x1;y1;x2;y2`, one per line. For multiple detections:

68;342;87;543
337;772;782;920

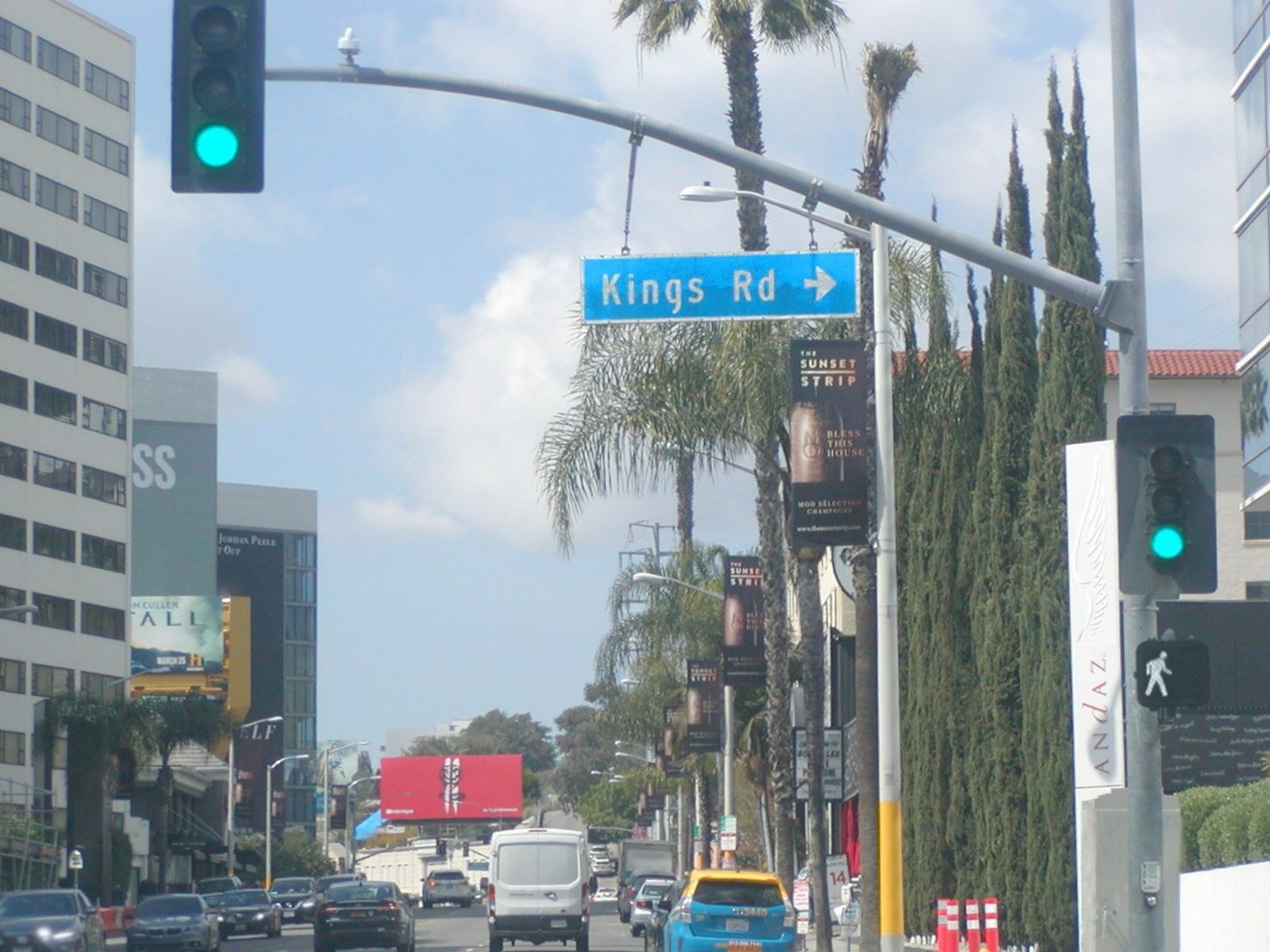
482;828;490;845
171;0;265;196
1110;412;1220;594
437;839;446;855
463;841;469;857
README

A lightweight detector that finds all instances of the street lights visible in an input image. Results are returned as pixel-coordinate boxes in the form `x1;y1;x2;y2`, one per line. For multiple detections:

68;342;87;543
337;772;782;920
654;438;762;482
101;666;173;904
227;716;284;877
264;754;310;889
587;566;738;867
0;604;39;613
344;775;382;875
682;181;908;951
322;740;371;862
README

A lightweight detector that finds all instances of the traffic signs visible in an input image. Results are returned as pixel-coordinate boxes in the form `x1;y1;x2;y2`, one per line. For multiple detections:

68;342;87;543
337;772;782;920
576;247;864;328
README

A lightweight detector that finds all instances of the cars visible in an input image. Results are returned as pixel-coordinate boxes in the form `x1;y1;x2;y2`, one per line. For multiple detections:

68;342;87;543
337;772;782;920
124;892;221;952
212;887;284;941
595;888;618;898
0;887;107;952
313;881;415;952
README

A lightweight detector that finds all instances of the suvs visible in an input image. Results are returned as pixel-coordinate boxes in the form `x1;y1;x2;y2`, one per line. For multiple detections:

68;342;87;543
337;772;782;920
419;870;473;907
617;868;799;952
194;877;245;908
592;858;617;876
268;873;369;925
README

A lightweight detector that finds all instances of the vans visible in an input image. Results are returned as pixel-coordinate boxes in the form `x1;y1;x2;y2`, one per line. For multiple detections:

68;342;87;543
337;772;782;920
615;836;679;923
489;827;597;952
